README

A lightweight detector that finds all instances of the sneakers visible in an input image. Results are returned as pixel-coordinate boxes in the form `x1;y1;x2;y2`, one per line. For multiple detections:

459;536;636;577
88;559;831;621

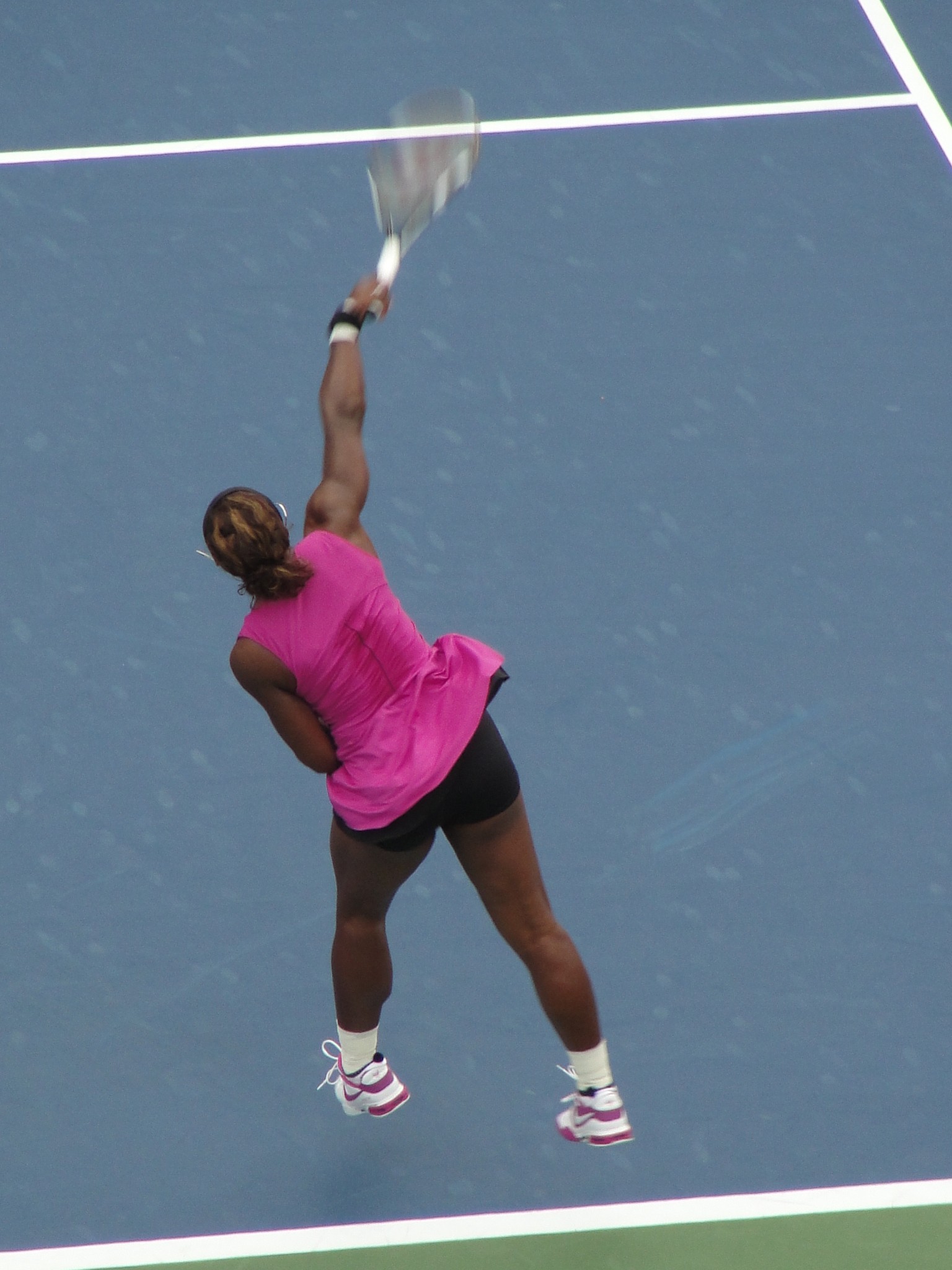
554;1063;636;1145
317;1038;409;1118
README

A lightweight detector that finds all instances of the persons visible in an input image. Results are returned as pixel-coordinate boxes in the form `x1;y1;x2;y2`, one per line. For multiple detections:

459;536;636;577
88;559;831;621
202;270;636;1147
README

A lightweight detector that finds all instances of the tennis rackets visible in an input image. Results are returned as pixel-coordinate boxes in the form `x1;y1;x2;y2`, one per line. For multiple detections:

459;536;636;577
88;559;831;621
358;86;479;325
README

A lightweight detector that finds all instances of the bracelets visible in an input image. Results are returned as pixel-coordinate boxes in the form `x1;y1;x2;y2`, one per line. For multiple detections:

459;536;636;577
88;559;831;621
328;297;366;345
